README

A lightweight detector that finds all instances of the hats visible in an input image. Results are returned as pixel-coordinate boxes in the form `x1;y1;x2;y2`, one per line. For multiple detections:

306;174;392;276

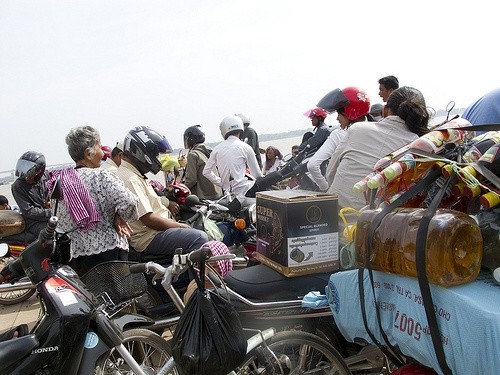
115;139;125;152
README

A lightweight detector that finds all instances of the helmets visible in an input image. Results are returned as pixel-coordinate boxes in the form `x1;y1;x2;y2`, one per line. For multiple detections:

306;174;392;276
123;125;173;176
100;145;113;160
234;113;251;127
219;115;244;139
184;126;205;149
309;108;327;118
15;150;46;181
332;87;370;120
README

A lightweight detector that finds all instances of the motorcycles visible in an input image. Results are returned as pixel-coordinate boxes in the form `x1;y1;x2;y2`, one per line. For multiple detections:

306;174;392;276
0;232;35;305
162;100;459;372
0;173;178;375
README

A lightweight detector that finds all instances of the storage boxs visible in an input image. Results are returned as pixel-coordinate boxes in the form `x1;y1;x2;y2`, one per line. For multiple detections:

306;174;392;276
255;189;341;278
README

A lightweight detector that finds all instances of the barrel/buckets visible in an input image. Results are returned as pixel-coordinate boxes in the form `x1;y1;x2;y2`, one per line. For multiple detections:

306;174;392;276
339;207;483;288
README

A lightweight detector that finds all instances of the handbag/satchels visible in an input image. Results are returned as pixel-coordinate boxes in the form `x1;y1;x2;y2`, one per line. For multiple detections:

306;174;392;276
171;260;247;375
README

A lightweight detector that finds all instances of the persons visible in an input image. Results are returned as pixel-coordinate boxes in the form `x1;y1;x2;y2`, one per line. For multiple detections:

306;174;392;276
0;195;23;215
307;86;386;192
202;114;282;198
117;125;210;304
99;141;124;170
11;150;55;241
461;86;500;138
262;146;285;176
234;113;263;171
292;107;332;157
327;86;436;240
183;240;233;307
378;75;401;103
45;125;140;279
183;125;223;201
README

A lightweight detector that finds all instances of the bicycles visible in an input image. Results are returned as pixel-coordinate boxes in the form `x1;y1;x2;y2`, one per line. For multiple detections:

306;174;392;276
94;245;351;375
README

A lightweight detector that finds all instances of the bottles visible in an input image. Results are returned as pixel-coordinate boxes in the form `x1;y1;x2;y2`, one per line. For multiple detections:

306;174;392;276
353;117;500;213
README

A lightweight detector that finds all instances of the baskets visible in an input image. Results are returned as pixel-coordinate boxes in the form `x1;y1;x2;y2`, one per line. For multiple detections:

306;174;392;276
80;261;148;306
